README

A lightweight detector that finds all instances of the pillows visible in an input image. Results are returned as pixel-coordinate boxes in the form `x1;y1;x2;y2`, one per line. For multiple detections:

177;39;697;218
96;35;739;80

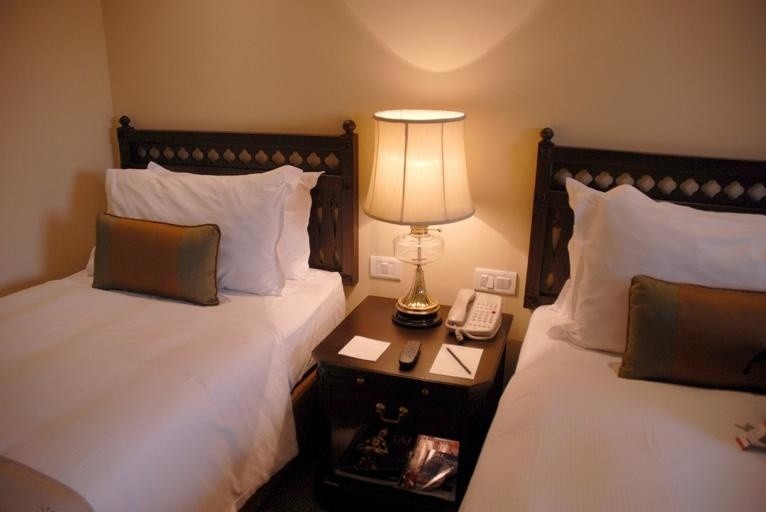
548;175;765;355
91;207;220;301
617;275;765;396
81;163;299;300
137;155;322;288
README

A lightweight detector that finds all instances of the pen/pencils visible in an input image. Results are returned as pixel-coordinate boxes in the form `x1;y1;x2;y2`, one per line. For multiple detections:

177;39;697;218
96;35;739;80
446;347;471;375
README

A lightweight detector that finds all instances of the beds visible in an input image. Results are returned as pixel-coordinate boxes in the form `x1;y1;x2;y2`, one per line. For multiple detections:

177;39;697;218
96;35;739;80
458;127;765;512
1;113;360;512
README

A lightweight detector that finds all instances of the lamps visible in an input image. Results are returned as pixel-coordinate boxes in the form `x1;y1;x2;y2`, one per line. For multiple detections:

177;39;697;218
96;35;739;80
364;106;477;331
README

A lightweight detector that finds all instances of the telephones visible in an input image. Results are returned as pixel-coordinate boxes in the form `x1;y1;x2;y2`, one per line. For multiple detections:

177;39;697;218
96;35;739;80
444;288;502;343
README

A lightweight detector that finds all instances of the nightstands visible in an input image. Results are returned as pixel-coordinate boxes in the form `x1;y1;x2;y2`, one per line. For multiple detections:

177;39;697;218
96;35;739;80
311;295;517;512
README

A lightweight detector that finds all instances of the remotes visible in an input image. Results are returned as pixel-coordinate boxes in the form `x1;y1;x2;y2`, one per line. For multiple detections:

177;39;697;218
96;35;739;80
397;339;423;367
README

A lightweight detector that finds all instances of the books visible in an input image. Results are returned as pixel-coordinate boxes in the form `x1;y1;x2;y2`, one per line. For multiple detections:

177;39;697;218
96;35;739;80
333;420;419;488
402;432;459;502
